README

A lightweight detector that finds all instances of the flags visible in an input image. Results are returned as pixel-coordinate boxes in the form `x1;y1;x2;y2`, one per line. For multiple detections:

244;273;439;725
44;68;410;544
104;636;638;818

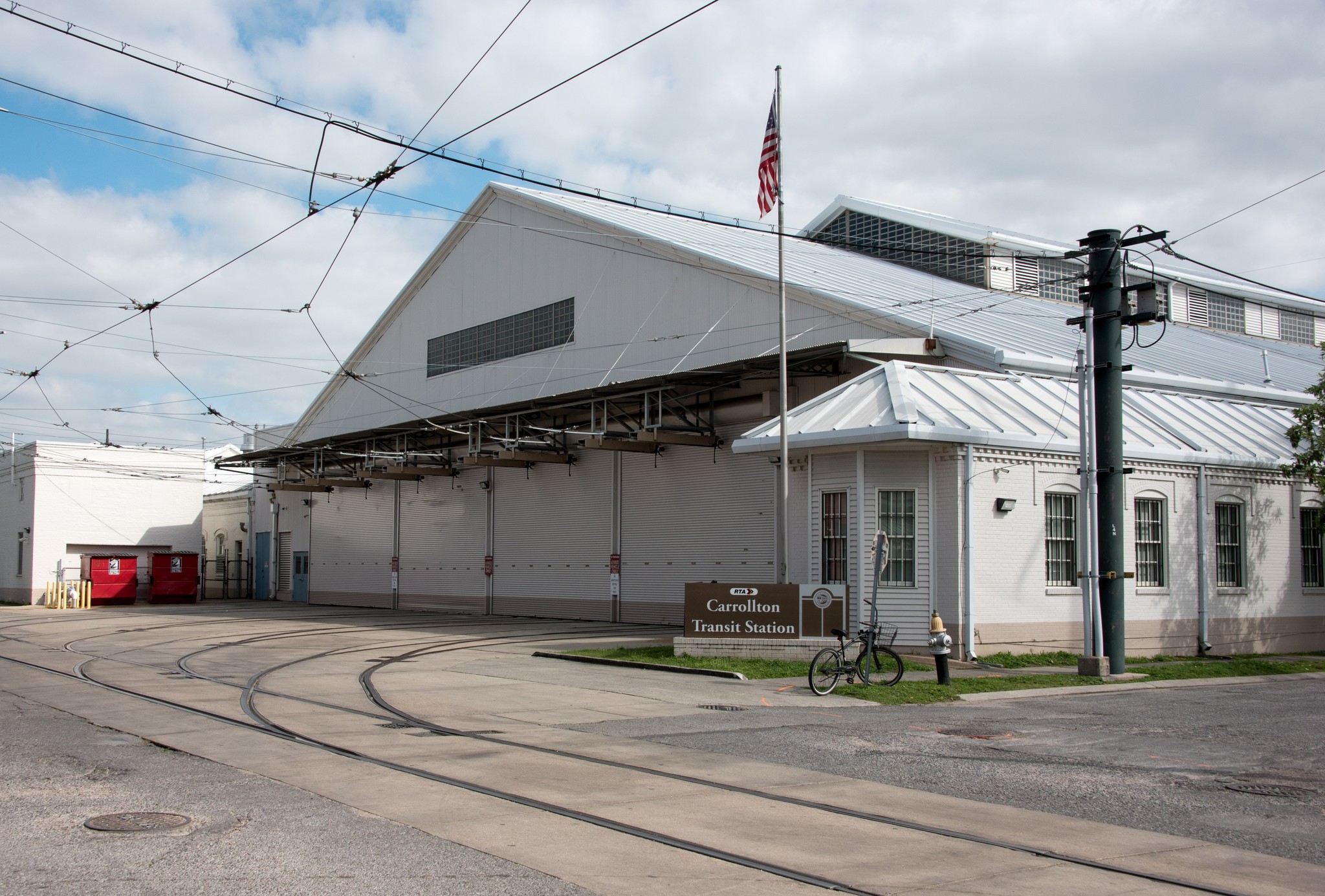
758;66;782;222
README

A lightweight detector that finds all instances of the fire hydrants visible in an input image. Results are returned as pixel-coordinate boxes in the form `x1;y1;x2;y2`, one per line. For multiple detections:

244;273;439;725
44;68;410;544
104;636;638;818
926;609;953;685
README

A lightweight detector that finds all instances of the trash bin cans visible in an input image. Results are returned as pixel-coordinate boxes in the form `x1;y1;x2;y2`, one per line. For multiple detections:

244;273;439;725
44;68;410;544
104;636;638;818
79;552;139;606
147;550;201;603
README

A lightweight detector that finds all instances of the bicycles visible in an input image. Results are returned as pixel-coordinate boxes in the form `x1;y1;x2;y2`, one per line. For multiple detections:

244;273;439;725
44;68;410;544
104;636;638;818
808;598;904;696
